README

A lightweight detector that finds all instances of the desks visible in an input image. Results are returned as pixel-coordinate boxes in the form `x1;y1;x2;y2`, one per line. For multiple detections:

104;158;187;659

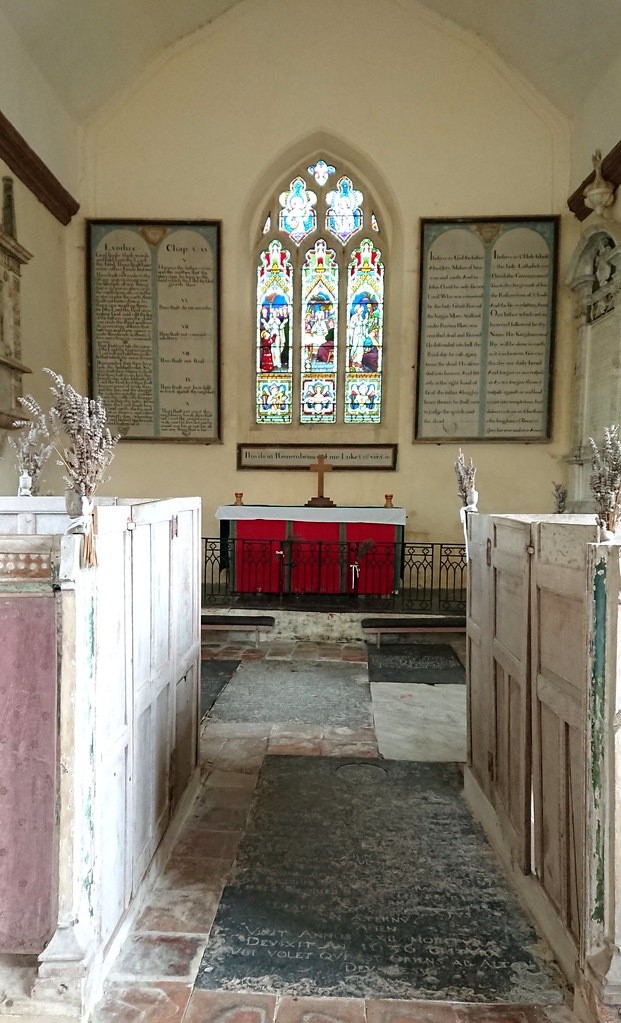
214;505;406;597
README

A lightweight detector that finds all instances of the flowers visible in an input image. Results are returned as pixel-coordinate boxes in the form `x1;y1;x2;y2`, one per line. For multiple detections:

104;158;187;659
549;478;570;516
449;444;478;507
584;422;621;533
4;366;123;570
